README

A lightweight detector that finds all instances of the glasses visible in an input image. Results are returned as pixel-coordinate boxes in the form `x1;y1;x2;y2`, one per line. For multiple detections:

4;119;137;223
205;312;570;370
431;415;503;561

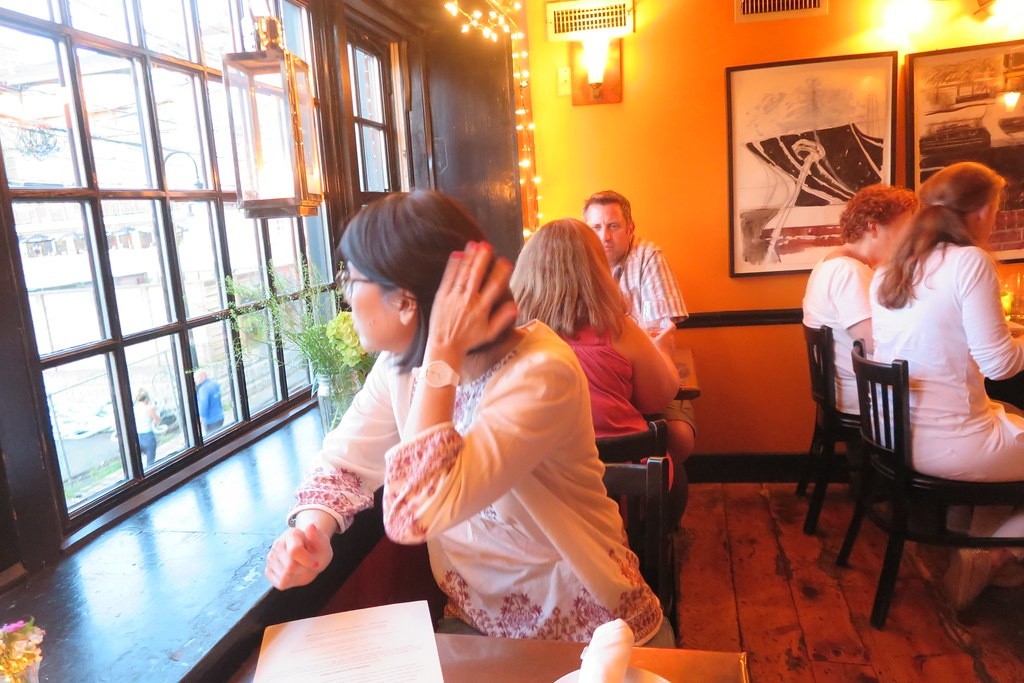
336;267;379;291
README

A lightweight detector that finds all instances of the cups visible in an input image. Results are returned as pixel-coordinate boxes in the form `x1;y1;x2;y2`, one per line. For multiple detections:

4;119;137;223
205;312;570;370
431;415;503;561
1002;272;1021;317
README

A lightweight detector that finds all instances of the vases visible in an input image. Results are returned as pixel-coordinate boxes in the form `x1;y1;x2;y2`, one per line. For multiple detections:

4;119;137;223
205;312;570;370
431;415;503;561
313;374;363;434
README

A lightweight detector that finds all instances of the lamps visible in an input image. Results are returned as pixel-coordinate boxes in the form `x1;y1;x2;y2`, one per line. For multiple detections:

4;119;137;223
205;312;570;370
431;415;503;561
222;15;323;217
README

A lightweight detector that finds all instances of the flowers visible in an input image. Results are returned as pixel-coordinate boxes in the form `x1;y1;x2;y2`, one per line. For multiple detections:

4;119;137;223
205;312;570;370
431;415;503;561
0;617;47;683
200;264;380;433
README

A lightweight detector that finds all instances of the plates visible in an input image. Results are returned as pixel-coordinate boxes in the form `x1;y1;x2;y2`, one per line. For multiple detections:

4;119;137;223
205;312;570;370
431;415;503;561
552;666;671;683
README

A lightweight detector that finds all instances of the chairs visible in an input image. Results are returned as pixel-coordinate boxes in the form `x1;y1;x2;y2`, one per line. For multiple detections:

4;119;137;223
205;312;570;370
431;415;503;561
596;420;669;555
602;458;674;618
795;319;863;537
834;337;1024;632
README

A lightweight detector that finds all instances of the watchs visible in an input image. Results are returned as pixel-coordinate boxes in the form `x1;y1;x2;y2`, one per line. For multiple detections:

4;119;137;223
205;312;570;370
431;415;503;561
412;359;461;387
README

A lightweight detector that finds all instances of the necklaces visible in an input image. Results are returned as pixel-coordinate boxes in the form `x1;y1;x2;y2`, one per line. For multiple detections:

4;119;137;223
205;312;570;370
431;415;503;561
463;355;479;385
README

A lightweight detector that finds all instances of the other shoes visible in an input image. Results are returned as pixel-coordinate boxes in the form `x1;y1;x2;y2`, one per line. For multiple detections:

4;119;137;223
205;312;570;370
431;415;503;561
943;550;992;608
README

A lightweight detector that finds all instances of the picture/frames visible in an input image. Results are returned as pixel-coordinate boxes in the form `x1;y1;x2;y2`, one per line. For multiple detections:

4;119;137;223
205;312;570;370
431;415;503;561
722;49;898;278
904;37;1024;265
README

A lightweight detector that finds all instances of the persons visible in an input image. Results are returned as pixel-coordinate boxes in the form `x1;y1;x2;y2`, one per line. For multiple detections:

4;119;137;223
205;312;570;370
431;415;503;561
194;368;224;434
266;189;694;652
802;162;1024;611
132;389;159;465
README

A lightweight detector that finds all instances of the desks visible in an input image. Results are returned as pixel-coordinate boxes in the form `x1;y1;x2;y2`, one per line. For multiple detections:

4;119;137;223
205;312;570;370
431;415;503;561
227;626;747;683
